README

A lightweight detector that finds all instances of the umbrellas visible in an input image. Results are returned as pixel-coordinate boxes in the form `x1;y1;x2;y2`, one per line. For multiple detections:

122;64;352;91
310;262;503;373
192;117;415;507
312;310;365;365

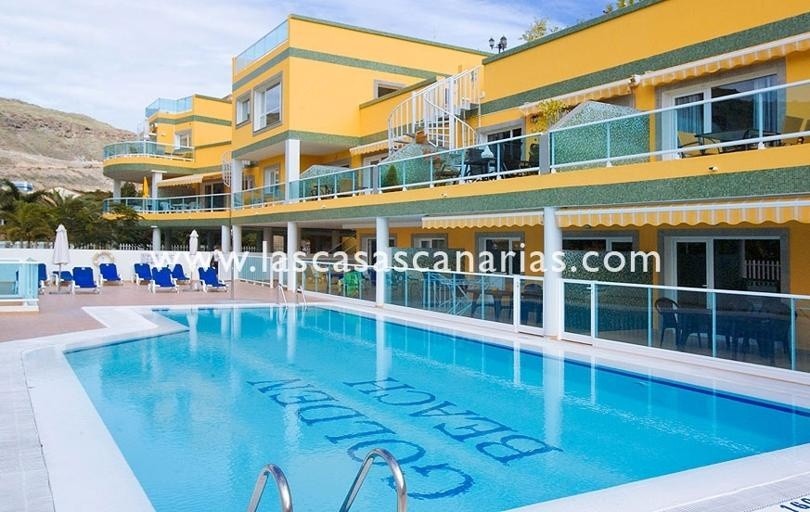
187;307;198;350
188;229;199;280
53;224;69;291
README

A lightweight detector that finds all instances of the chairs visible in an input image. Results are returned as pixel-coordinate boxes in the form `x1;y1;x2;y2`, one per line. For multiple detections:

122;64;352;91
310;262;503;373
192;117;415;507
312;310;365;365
160;201;202;214
434;164;461;187
520;143;539;175
14;259;230;297
464;149;490;180
521;283;543;324
655;287;797;367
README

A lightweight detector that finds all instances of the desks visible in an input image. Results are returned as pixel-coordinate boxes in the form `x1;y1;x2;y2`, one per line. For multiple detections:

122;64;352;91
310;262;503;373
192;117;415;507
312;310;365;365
694;127;780;153
466;288;516;321
